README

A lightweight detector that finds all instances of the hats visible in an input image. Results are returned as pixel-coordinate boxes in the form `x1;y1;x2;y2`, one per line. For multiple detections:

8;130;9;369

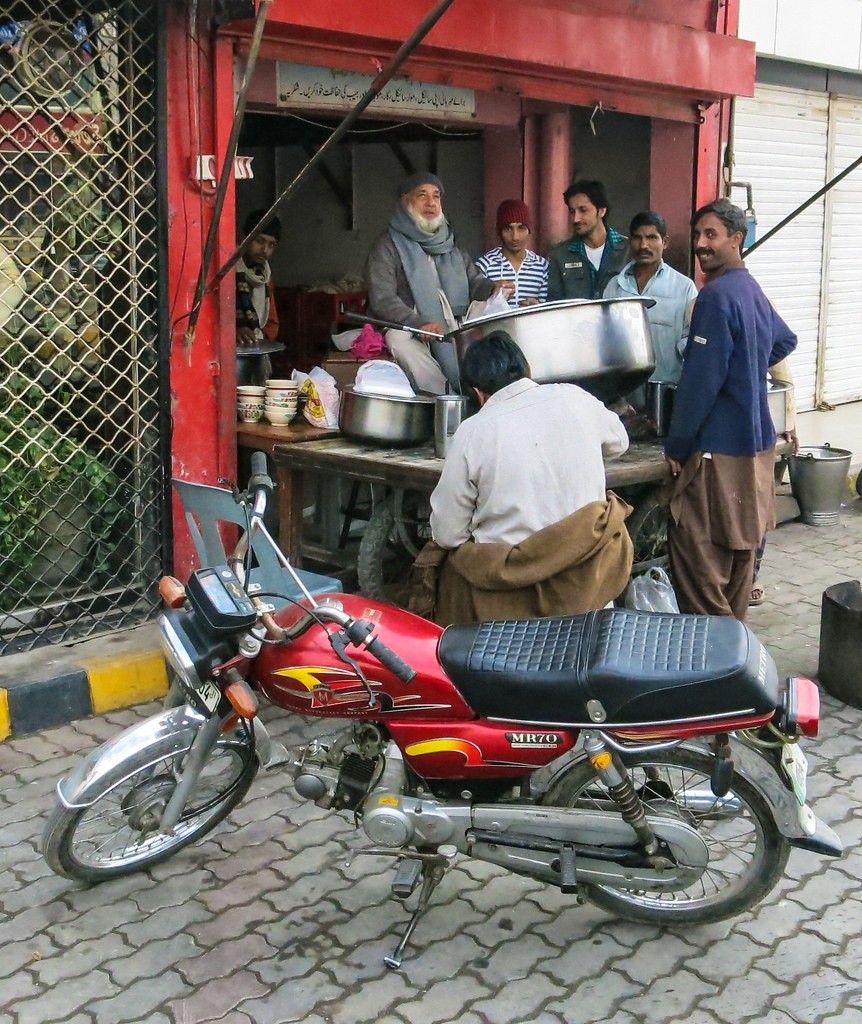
496;199;532;236
245;209;282;241
397;172;444;198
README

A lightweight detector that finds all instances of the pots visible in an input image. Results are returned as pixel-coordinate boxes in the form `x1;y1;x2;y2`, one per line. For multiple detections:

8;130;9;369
449;296;657;407
766;380;793;435
338;385;440;447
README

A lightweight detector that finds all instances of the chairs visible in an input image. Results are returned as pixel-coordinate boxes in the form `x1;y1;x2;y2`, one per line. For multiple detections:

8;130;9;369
173;478;343;615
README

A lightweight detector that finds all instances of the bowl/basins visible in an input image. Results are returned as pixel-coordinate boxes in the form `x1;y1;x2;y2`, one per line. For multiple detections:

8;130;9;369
238;379;298;426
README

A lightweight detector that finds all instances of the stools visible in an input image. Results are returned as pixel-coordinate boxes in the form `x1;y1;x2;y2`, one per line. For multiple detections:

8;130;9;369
817;580;862;710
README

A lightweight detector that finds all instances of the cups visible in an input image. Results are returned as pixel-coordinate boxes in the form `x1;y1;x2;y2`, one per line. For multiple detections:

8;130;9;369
434;395;468;459
644;380;671;438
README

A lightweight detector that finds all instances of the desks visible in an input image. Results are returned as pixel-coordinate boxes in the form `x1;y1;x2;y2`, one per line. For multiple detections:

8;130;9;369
239;420;341;566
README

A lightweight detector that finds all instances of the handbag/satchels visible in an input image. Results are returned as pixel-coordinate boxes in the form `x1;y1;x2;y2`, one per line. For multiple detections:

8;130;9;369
292;366;341;429
625;567;681;615
353;360;417;397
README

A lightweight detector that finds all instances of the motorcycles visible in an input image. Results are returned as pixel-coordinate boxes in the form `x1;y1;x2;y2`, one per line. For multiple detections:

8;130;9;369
36;451;850;967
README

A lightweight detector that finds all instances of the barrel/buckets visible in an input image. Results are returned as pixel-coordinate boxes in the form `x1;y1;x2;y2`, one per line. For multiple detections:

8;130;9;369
788;443;851;526
236;353;265;385
819;580;862;711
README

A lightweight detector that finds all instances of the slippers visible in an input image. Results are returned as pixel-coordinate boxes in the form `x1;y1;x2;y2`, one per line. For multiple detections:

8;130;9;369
747;582;765;605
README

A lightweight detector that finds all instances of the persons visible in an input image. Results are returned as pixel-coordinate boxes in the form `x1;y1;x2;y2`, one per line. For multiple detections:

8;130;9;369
235;210;279;385
603;211;699;410
664;198;798;620
474;199;549;309
542;179;629;303
408;329;634;625
362;171;516;394
